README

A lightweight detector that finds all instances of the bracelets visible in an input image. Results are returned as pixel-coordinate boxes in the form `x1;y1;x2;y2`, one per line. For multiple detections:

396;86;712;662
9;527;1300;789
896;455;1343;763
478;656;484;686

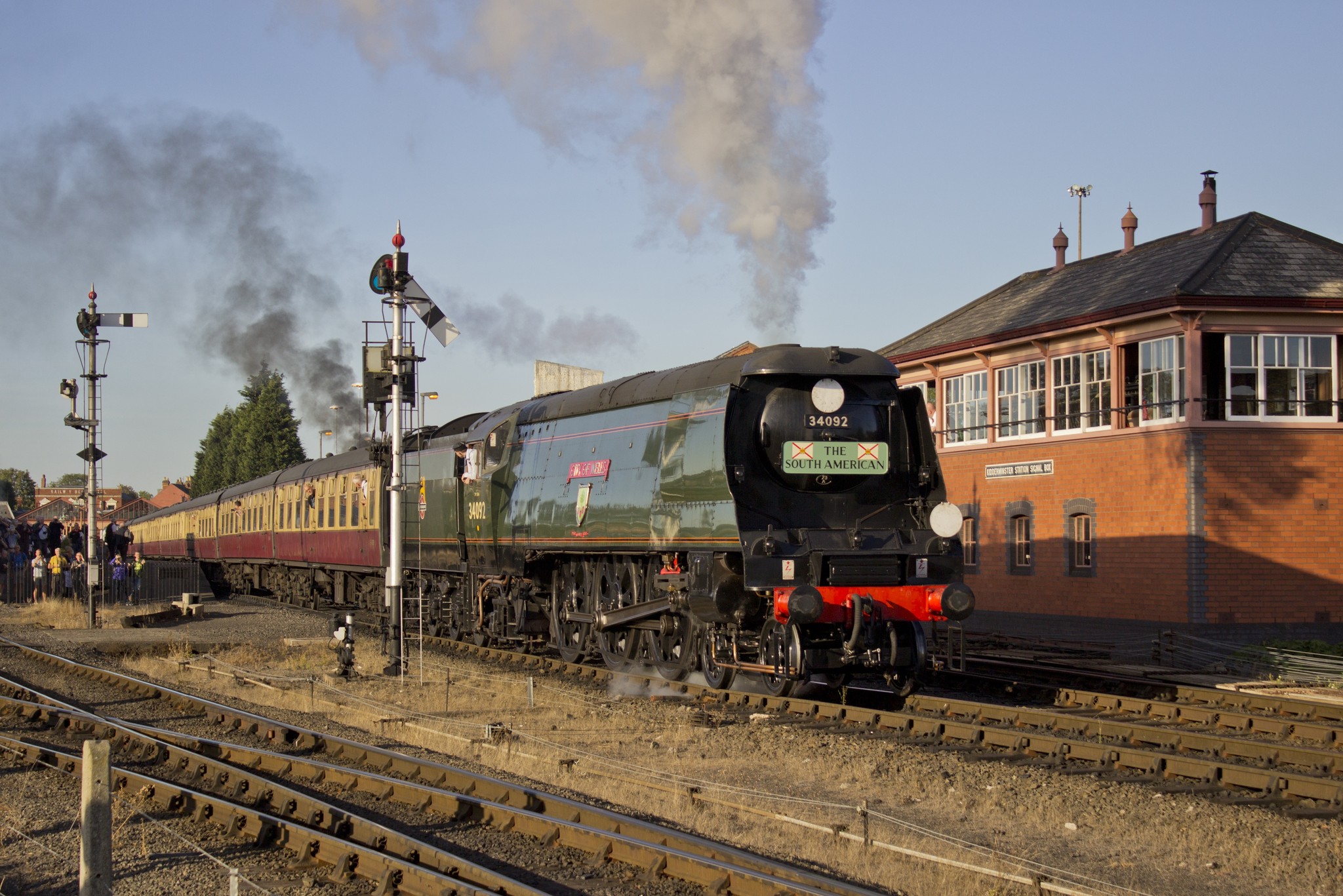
2;541;3;543
24;568;27;570
16;532;18;534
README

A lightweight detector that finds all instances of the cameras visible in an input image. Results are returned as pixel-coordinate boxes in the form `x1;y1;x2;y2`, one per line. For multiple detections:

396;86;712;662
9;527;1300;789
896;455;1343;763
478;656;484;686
231;508;237;511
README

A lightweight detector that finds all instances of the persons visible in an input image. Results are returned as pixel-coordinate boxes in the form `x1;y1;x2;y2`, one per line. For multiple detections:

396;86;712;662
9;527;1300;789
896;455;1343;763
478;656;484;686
303;484;316;509
192;513;201;527
452;442;498;485
235;501;245;518
353;475;368;506
926;403;952;444
0;516;146;605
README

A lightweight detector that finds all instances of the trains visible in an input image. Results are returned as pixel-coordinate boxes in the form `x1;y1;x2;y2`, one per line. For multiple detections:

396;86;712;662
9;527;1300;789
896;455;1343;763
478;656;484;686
99;342;977;714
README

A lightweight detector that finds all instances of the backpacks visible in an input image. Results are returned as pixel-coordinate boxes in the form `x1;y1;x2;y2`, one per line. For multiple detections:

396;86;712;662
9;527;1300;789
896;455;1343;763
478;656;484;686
124;529;134;543
39;525;48;540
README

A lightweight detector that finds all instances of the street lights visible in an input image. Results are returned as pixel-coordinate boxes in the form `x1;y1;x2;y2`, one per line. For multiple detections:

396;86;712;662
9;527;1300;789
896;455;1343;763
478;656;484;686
1068;184;1092;261
420;391;439;433
58;490;87;538
330;406;343;455
351;383;363;448
319;430;333;458
